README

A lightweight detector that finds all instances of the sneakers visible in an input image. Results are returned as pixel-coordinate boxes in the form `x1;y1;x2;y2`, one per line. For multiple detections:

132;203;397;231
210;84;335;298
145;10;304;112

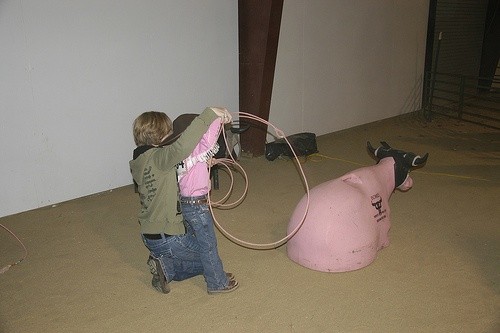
203;271;235;281
205;279;239;294
148;255;157;279
148;255;171;295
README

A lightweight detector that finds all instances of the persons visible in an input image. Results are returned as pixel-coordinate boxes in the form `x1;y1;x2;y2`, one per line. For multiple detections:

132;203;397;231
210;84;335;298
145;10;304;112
173;113;239;294
129;107;232;293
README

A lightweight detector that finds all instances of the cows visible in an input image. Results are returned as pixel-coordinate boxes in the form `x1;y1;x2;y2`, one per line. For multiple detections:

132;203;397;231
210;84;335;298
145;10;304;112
286;141;429;273
